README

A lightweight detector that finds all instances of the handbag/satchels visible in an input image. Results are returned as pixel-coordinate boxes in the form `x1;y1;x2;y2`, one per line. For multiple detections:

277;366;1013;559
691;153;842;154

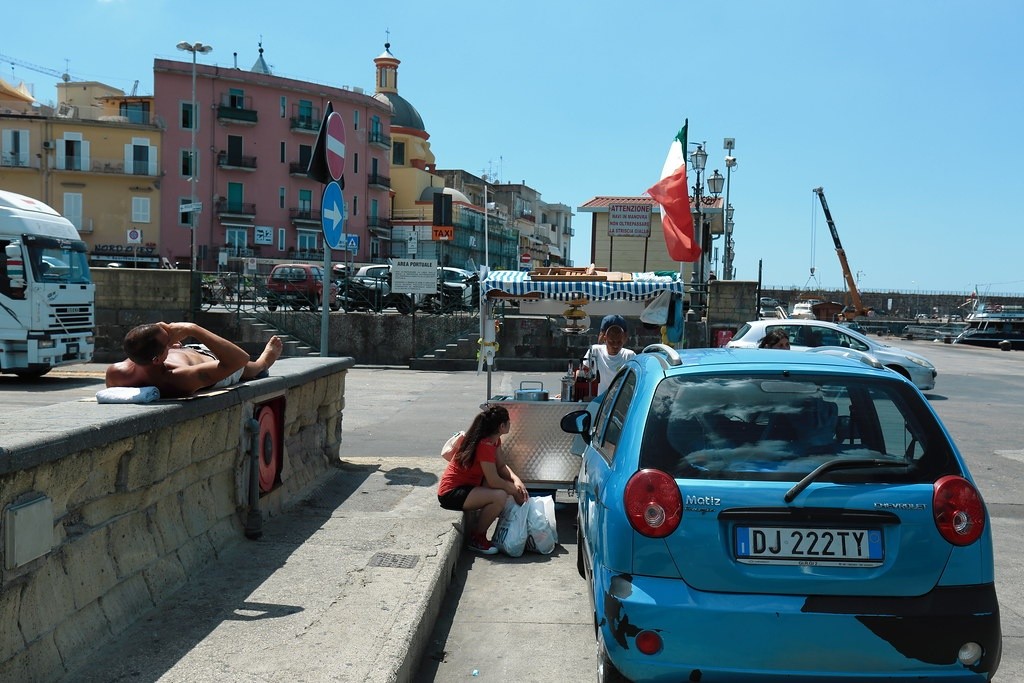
528;499;555;555
441;430;467;461
492;496;528;558
638;291;684;344
541;495;559;545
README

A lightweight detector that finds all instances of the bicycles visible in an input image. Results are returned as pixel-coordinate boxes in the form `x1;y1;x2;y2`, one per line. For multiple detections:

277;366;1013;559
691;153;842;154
202;274;242;314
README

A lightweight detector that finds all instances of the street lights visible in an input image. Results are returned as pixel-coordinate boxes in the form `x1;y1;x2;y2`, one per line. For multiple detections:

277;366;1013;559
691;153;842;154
723;202;734;282
686;145;726;322
176;41;212;274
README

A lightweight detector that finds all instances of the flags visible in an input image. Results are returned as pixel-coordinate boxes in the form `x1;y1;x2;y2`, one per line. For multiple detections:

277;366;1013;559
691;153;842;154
645;120;703;263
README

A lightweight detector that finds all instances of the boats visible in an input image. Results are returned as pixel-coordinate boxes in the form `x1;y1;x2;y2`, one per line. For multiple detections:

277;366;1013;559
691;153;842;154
901;286;1024;349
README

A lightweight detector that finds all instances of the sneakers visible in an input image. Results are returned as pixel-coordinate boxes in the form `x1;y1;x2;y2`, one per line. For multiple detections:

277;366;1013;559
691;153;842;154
468;537;498;554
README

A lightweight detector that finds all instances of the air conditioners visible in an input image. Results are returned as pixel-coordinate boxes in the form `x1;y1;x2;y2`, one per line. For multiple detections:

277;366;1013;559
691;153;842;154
42;141;54;149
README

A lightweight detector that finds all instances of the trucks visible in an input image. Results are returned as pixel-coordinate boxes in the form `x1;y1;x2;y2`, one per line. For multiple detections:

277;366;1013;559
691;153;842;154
0;191;96;379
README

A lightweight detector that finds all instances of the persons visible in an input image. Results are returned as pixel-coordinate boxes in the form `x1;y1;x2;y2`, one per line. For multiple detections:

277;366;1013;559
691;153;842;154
758;329;790;349
581;315;637;395
106;321;283;394
437;405;529;559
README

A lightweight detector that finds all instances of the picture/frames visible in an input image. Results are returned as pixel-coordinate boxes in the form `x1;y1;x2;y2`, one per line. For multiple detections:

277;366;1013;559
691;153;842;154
254;225;274;245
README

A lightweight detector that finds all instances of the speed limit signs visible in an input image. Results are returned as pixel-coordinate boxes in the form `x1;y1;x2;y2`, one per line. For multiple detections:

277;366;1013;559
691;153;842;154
521;254;531;264
323;111;348;179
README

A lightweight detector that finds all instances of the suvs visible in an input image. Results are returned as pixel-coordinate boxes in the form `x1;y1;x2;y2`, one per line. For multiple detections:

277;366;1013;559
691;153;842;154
332;262;413;314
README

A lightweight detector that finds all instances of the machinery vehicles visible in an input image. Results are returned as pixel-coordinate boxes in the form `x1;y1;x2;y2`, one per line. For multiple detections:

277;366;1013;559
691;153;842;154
799;185;883;321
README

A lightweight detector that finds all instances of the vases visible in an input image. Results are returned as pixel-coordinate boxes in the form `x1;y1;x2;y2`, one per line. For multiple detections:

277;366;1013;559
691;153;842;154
299;122;306;128
218;202;228;212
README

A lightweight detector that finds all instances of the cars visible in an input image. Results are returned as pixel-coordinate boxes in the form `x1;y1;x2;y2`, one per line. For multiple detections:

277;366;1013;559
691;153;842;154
725;320;937;394
266;263;340;312
354;264;418;304
421;266;480;309
558;343;1002;683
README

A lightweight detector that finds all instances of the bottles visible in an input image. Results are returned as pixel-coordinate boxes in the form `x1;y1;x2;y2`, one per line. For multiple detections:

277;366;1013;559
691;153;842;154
575;376;587;402
591;374;598;397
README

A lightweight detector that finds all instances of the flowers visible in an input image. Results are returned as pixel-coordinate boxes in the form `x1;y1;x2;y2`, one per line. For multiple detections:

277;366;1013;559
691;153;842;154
288;246;295;251
224;241;233;248
246;243;253;249
218;149;227;154
310;248;318;253
219;196;227;201
299;247;307;252
